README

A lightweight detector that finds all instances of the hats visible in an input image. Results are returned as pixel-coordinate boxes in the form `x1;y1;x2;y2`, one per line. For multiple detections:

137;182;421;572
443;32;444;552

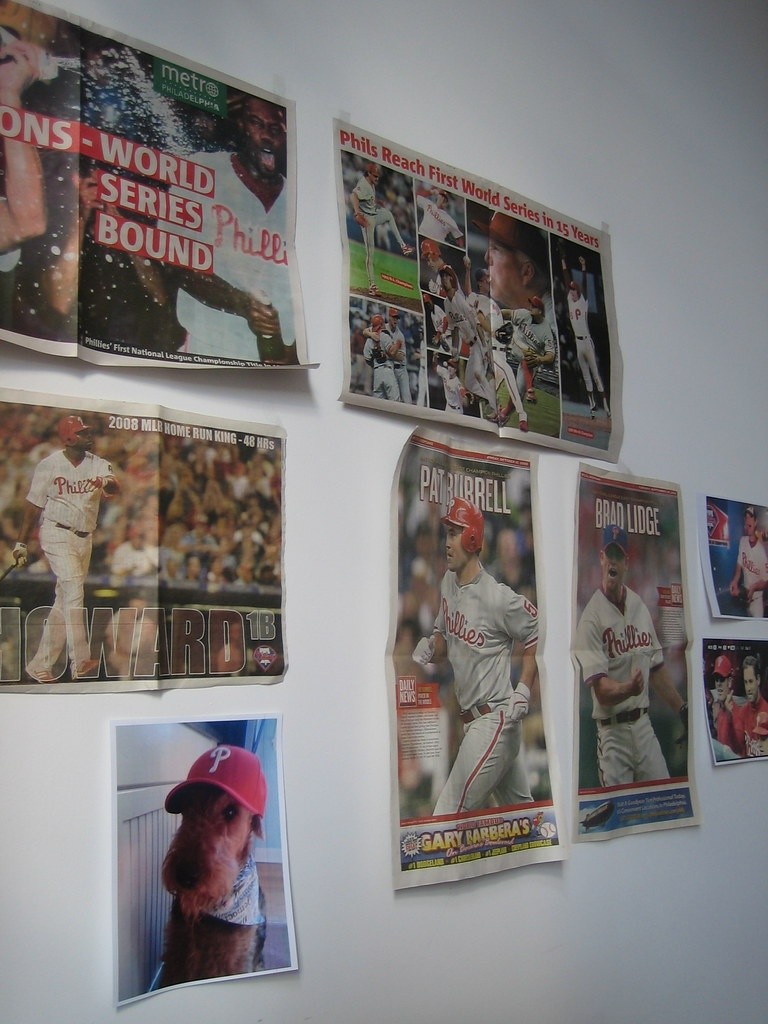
369;165;381;179
475;269;490;281
528;296;544;310
752;712;768;735
569;281;581;298
712;656;732;677
745;507;757;518
439;189;449;202
472;211;541;262
601;524;628;557
165;745;267;818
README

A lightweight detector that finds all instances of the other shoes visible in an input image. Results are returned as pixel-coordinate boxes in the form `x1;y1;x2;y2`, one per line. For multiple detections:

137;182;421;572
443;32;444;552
591;406;597;418
607;411;612;420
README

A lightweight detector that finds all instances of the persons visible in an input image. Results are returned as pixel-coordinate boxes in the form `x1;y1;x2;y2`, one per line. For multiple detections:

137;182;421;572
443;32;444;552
0;0;768;821
412;496;540;817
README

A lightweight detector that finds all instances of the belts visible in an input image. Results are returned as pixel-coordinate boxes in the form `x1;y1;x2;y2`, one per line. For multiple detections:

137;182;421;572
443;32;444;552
601;708;648;726
461;705;490;723
374;337;586;410
57;522;91;538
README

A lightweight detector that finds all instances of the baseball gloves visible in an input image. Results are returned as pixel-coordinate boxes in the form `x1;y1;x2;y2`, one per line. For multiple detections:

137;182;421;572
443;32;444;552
353;211;370;227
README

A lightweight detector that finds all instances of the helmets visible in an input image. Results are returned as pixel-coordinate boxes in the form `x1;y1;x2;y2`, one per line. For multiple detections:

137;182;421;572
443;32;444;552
438;496;483;556
420;240;441;258
58;416;92;443
423;293;431;303
438;265;458;290
389;307;400;320
371;314;383;327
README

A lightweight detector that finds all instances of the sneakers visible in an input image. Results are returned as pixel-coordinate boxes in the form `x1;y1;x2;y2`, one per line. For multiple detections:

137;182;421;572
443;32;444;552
70;658;102;680
403;244;417;256
25;662;58;684
496;389;537;432
369;284;382;297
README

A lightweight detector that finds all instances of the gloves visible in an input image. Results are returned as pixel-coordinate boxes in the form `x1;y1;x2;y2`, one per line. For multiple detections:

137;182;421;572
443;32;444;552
429;279;438;292
411;636;436;666
83;476;117;495
504;681;532;722
11;542;28;568
579;256;586;271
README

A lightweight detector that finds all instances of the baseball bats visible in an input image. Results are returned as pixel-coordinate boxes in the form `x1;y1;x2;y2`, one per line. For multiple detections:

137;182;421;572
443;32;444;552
0;552;24;580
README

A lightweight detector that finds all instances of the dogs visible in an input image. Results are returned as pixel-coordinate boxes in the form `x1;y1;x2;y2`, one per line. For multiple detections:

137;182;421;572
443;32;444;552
162;744;266;985
